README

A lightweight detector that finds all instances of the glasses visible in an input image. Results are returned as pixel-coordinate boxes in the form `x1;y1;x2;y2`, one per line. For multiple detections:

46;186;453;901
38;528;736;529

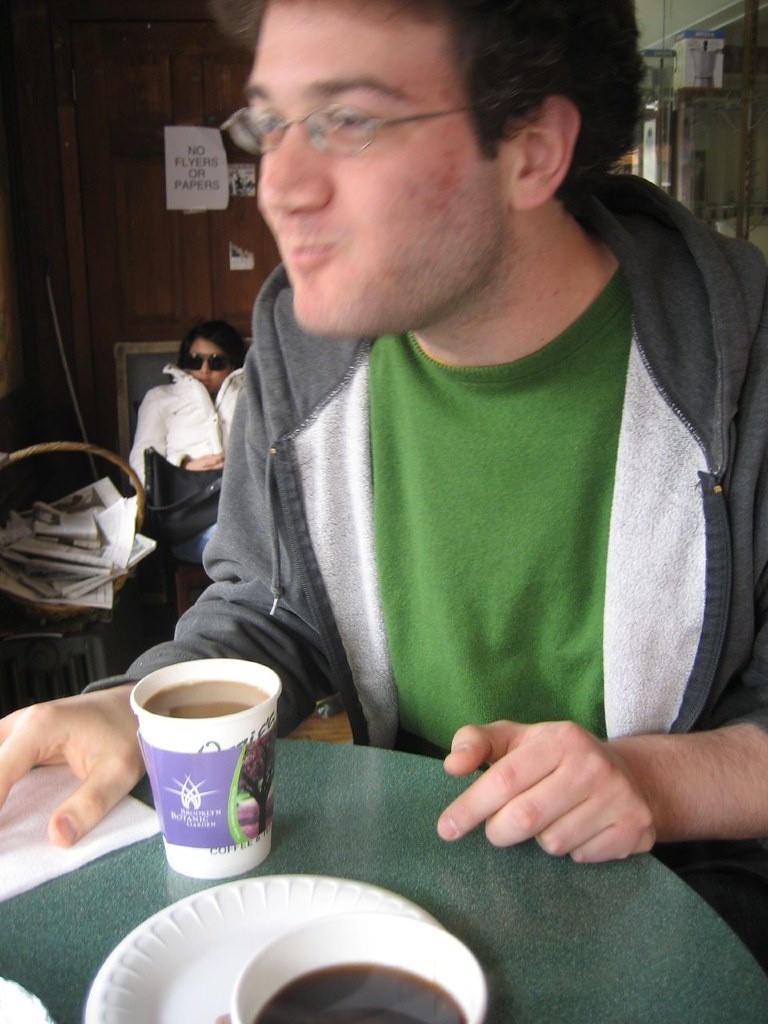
178;352;233;371
218;105;485;157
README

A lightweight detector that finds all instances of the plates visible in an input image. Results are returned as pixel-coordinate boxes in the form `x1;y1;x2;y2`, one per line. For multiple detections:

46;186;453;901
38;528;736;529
84;873;443;1024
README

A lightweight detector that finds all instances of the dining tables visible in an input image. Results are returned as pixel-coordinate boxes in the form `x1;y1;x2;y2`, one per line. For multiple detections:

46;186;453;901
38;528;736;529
0;741;768;1024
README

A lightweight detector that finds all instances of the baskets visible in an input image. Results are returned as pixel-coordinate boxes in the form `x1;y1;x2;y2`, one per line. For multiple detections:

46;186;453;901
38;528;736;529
0;443;146;623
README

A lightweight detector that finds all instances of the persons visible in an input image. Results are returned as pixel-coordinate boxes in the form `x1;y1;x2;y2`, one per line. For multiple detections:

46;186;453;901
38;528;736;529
129;320;245;566
0;0;768;865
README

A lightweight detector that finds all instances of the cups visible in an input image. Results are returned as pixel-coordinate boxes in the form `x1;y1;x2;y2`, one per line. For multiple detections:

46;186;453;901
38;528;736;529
233;911;488;1024
129;657;282;877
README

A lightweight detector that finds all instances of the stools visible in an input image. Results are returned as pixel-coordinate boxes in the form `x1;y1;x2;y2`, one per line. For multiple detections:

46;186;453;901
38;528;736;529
0;583;138;720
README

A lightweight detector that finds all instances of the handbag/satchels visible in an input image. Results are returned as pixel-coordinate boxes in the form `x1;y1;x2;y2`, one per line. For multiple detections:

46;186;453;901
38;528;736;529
137;448;225;545
